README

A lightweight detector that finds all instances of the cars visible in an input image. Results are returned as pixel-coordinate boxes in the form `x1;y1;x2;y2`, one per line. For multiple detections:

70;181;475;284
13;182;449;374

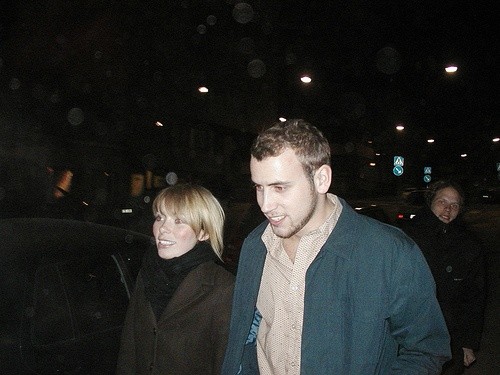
1;217;240;375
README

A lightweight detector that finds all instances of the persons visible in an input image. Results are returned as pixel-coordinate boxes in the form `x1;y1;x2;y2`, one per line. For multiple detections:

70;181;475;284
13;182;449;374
394;182;484;375
220;116;453;375
117;183;237;374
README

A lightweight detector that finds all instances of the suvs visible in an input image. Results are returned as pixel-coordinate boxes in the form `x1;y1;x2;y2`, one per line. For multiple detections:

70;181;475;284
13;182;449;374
396;189;436;226
